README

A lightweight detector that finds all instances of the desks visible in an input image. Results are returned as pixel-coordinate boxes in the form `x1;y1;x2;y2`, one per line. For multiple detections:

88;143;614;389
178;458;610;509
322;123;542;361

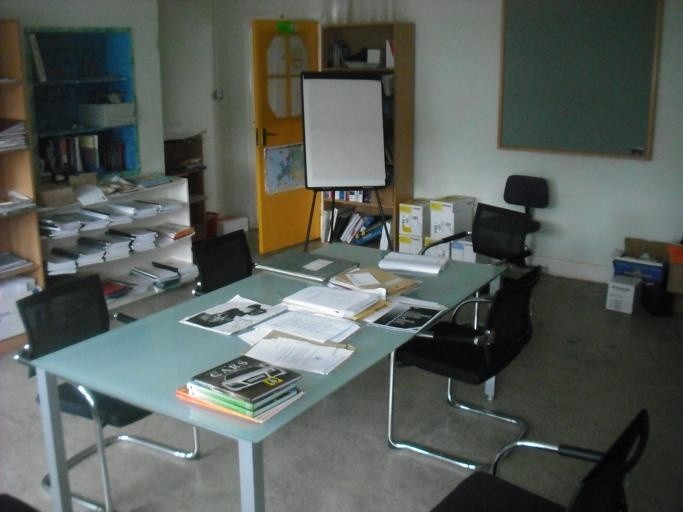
27;240;509;512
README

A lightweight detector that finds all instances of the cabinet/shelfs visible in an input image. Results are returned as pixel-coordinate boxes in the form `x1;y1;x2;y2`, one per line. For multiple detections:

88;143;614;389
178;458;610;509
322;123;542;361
0;18;201;343
315;20;415;252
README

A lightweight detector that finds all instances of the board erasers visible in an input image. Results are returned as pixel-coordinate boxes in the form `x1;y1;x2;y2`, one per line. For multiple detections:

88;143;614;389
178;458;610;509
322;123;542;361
630;148;644;155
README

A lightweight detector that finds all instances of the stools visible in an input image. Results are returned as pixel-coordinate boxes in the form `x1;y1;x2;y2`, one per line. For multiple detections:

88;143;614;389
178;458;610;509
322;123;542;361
502;174;552;258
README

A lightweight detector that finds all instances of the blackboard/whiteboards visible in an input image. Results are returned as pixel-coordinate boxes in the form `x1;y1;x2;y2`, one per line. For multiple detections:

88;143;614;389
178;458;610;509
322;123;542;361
496;0;662;161
301;71;388;189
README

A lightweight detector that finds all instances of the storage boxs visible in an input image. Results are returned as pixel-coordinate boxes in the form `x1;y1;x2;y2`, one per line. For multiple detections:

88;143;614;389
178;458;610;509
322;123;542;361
397;194;492;264
604;236;683;315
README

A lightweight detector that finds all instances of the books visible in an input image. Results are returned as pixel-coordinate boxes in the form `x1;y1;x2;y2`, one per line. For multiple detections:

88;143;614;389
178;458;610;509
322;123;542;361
0;193;33;213
0;118;27;149
321;38;395;253
30;32;47;82
0;251;29;273
37;133;126;182
174;248;450;423
40;197;199;299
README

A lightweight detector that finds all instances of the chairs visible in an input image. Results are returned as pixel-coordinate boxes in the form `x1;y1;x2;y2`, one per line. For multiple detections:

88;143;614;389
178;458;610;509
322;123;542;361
187;227;255;294
425;408;646;511
388;265;542;473
11;272;201;510
416;203;530;332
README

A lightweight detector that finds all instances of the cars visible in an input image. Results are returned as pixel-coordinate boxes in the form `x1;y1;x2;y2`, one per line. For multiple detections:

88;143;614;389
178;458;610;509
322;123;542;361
220;365;288;392
